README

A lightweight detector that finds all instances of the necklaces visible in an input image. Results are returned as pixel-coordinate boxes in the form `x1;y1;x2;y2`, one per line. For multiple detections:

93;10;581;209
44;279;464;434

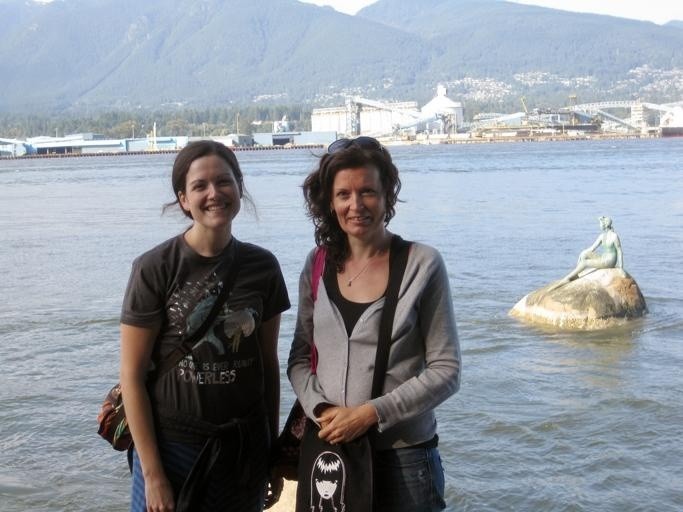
338;244;387;288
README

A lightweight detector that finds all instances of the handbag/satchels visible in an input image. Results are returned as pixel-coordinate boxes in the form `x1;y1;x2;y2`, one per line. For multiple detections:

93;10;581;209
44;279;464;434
97;383;137;451
294;420;380;512
278;396;309;481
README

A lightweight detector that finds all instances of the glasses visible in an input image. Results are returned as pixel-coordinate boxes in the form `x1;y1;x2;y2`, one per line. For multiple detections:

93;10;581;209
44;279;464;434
325;136;383;154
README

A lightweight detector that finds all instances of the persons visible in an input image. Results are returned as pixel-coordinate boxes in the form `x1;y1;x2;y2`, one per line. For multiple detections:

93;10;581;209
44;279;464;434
546;216;623;293
286;137;462;512
119;140;290;512
